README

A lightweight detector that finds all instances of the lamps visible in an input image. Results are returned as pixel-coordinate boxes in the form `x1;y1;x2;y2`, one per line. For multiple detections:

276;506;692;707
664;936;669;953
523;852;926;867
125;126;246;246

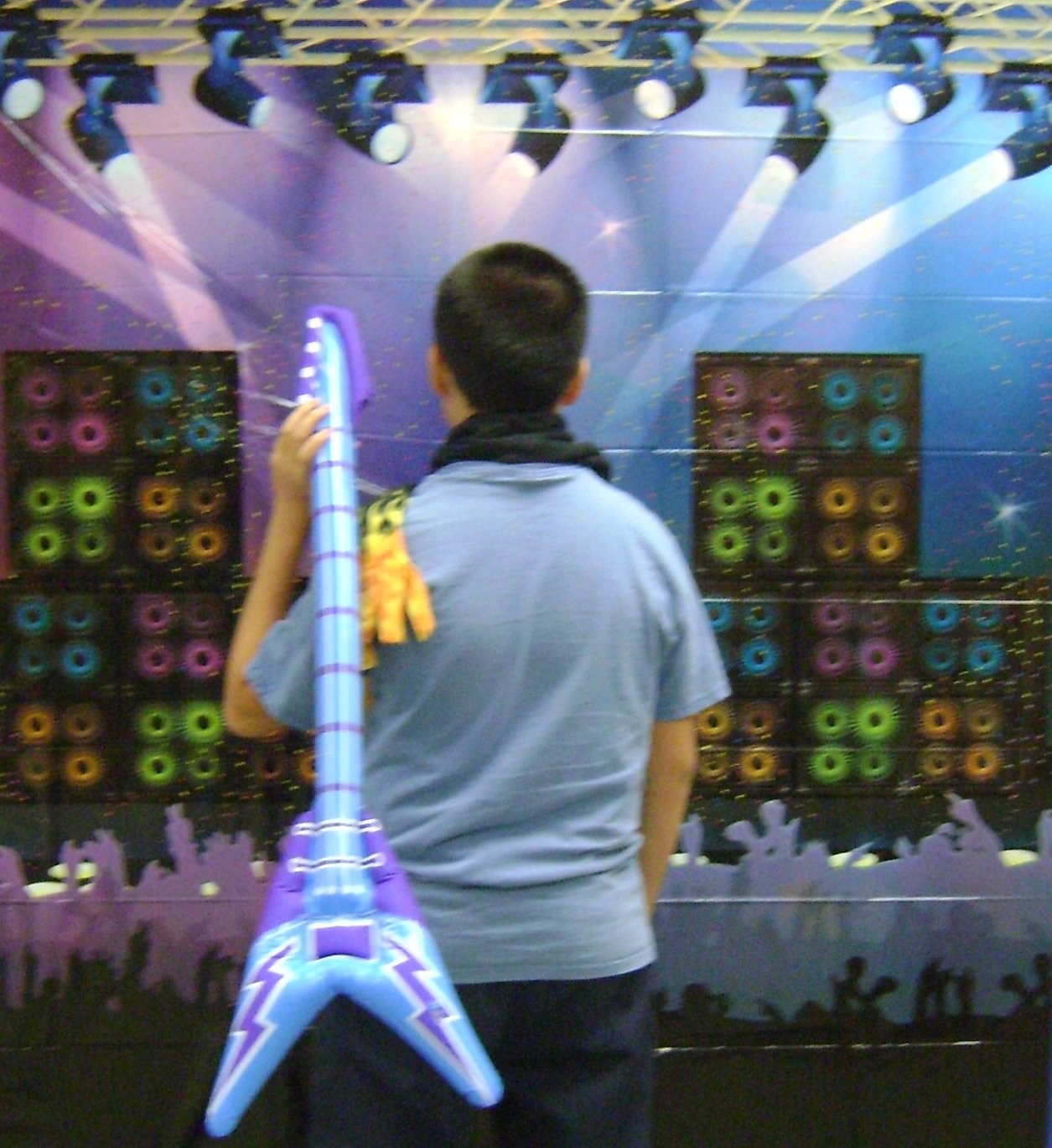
0;0;1051;184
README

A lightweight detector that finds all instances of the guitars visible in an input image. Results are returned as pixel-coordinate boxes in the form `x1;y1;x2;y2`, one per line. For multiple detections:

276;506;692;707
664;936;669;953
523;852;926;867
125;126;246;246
202;297;502;1140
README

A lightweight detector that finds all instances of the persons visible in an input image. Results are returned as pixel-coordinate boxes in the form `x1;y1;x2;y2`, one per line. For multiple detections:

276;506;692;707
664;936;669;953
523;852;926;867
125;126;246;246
221;242;729;1148
